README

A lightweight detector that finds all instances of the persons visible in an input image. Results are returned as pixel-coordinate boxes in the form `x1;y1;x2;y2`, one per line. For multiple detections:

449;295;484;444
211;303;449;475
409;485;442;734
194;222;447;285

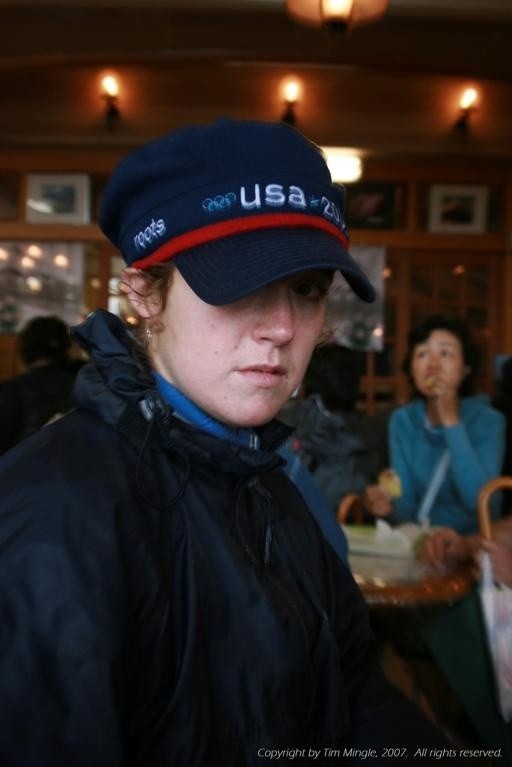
0;315;89;455
276;312;512;767
0;119;462;767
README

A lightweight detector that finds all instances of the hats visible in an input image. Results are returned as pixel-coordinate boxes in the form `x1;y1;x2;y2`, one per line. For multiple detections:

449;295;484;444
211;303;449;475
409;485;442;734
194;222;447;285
98;118;377;306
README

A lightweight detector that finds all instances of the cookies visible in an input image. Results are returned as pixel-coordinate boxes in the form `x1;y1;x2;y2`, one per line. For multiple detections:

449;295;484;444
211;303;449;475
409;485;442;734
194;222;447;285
379;470;401;496
425;374;435;387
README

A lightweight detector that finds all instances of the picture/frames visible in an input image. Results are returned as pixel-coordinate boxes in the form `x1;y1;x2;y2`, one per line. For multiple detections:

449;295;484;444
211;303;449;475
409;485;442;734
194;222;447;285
422;180;495;235
20;170;95;225
